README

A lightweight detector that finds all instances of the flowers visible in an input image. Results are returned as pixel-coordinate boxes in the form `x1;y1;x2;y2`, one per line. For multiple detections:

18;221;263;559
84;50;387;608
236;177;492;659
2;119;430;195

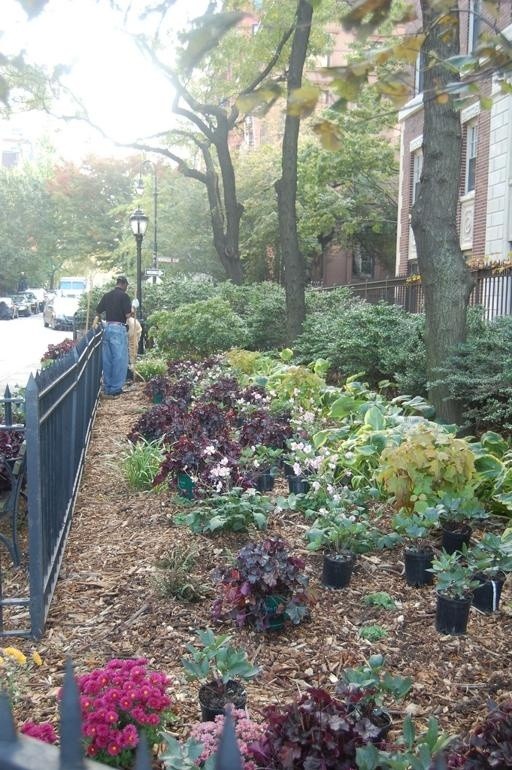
2;644;44;671
184;708;271;769
60;659;174;759
139;351;475;554
20;718;56;750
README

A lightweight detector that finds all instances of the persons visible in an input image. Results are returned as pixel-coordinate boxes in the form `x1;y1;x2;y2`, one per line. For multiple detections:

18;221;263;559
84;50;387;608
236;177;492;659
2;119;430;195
95;276;143;396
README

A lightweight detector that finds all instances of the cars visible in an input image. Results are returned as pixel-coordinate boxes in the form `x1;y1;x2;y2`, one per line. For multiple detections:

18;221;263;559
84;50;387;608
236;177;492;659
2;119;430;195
0;284;87;331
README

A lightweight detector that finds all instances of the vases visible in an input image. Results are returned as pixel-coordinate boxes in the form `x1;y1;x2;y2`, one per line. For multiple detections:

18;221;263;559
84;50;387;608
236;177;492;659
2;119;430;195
321;548;355;590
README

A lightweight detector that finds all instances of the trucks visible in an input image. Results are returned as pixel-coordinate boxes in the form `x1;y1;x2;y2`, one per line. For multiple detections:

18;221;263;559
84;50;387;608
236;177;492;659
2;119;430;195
58;275;88;298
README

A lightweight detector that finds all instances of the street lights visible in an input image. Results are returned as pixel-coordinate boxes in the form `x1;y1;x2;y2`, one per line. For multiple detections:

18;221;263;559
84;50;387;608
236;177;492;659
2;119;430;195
128;202;151;358
135;158;159;287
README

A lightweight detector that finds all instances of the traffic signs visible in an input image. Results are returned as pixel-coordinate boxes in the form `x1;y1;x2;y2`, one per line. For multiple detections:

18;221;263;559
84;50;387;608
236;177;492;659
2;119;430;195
144;267;166;277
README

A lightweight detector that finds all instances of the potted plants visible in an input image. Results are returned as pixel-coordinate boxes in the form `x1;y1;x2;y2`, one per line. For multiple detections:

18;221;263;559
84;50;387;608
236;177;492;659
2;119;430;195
380;491;438;586
424;545;474;636
462;530;511;613
327;651;414;749
210;534;312;631
178;623;263;727
